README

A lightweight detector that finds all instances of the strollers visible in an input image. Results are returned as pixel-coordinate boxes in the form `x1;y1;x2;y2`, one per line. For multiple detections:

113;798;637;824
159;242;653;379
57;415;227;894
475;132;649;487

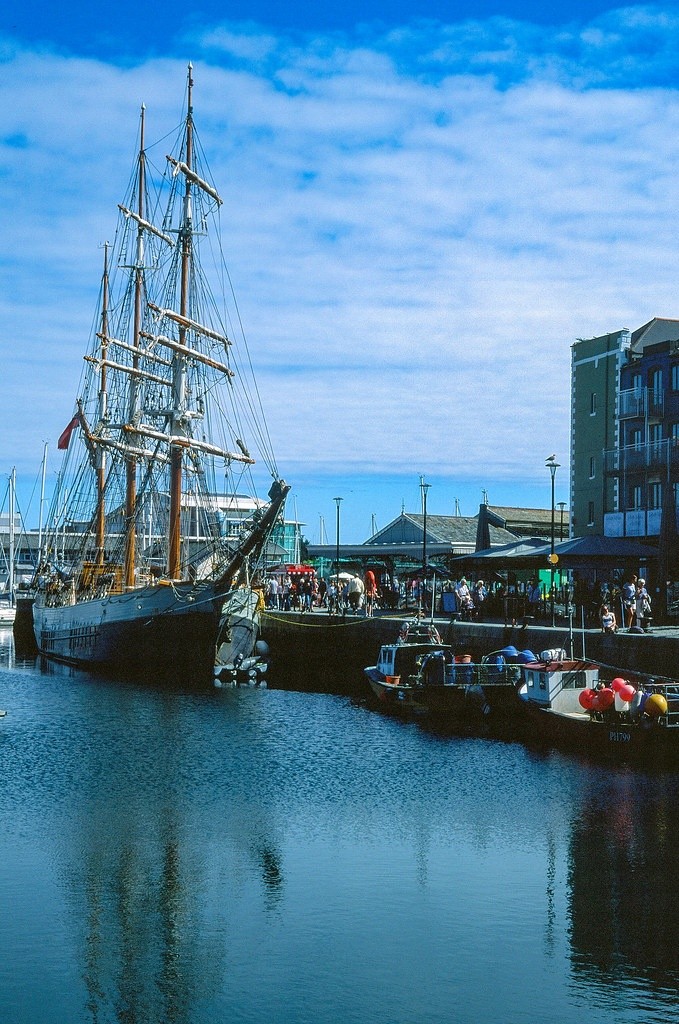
456;594;483;622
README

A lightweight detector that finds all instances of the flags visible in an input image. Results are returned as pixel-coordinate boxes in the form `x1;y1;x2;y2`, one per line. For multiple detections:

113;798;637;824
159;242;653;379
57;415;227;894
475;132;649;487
58;411;79;450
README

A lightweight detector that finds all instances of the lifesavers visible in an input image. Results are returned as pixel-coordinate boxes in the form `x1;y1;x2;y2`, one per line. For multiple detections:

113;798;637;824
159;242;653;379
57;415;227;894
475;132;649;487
425;624;444;646
397;621;412;644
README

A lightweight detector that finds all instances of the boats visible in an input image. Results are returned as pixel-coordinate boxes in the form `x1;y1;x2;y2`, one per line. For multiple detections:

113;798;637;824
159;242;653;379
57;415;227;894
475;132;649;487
361;613;497;724
494;644;679;762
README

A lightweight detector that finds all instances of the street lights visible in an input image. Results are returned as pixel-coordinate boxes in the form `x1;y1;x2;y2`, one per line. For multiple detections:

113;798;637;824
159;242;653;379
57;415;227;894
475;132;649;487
416;481;438;609
541;456;567;626
327;494;345;612
557;498;567;577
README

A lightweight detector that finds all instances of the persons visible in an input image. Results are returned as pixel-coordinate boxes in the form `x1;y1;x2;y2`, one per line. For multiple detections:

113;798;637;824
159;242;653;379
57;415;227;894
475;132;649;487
264;574;327;612
599;604;619;634
327;571;378;617
379;573;622;622
622;574;637;628
635;578;648;627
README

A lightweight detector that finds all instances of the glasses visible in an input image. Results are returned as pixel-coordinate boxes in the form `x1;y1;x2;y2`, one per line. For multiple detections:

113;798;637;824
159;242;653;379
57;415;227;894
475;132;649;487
604;608;608;611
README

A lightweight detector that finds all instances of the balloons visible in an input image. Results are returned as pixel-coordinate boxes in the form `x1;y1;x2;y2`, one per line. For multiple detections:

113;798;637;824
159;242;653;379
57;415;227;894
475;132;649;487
579;678;637;711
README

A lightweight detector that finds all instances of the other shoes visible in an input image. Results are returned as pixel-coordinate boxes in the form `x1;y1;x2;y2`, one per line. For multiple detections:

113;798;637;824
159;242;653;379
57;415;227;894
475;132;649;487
369;614;373;617
352;609;358;615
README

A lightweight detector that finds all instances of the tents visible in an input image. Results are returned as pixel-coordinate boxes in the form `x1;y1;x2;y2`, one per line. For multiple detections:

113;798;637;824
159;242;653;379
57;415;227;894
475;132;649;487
329;572;355;593
397;564;447;616
265;563;315;593
509;533;664;629
451;538;554;621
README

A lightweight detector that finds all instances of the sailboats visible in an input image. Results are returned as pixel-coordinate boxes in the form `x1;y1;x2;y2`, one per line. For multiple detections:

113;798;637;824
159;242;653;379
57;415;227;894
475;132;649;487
11;63;296;696
0;466;42;630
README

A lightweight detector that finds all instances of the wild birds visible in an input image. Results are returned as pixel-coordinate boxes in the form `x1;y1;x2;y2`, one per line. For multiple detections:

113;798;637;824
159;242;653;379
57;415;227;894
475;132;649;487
545;454;558;465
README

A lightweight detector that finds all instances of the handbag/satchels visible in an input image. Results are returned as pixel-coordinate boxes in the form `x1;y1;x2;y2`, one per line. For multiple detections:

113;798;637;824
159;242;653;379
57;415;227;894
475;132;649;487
312;594;317;600
